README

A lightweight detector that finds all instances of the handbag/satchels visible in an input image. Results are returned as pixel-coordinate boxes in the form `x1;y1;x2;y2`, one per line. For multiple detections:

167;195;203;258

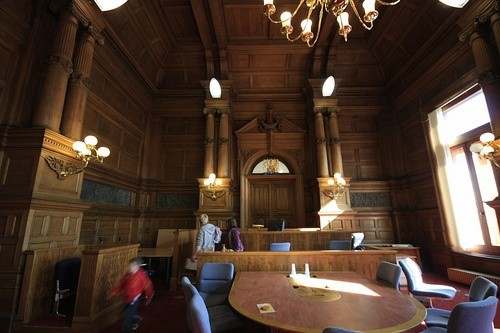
213;226;222;243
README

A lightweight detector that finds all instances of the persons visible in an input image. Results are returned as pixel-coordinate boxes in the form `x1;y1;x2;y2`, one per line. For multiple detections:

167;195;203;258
225;219;244;252
106;258;153;333
195;214;217;252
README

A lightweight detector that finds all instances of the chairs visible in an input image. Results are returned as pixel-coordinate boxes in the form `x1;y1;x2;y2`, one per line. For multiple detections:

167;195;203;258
180;262;243;333
270;241;291;252
49;256;81;317
328;233;364;249
268;218;286;231
377;257;499;333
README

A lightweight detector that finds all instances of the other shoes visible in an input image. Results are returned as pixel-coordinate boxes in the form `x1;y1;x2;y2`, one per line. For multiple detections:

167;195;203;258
131;316;142;330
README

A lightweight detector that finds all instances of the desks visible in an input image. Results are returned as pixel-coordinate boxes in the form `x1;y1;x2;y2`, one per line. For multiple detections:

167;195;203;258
197;250;398;278
383;246;422;285
138;247;173;280
228;270;428;333
240;230;362;250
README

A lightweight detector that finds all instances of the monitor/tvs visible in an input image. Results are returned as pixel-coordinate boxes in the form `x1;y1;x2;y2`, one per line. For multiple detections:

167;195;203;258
350;232;365;250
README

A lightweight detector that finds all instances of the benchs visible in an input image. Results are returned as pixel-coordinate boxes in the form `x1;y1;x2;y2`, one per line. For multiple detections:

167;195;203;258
447;267;500;293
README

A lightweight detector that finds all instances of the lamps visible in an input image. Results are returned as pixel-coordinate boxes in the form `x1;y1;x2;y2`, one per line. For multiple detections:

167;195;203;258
73;134;110;175
469;132;500;169
327;173;347;200
262;0;401;47
203;172;222;201
